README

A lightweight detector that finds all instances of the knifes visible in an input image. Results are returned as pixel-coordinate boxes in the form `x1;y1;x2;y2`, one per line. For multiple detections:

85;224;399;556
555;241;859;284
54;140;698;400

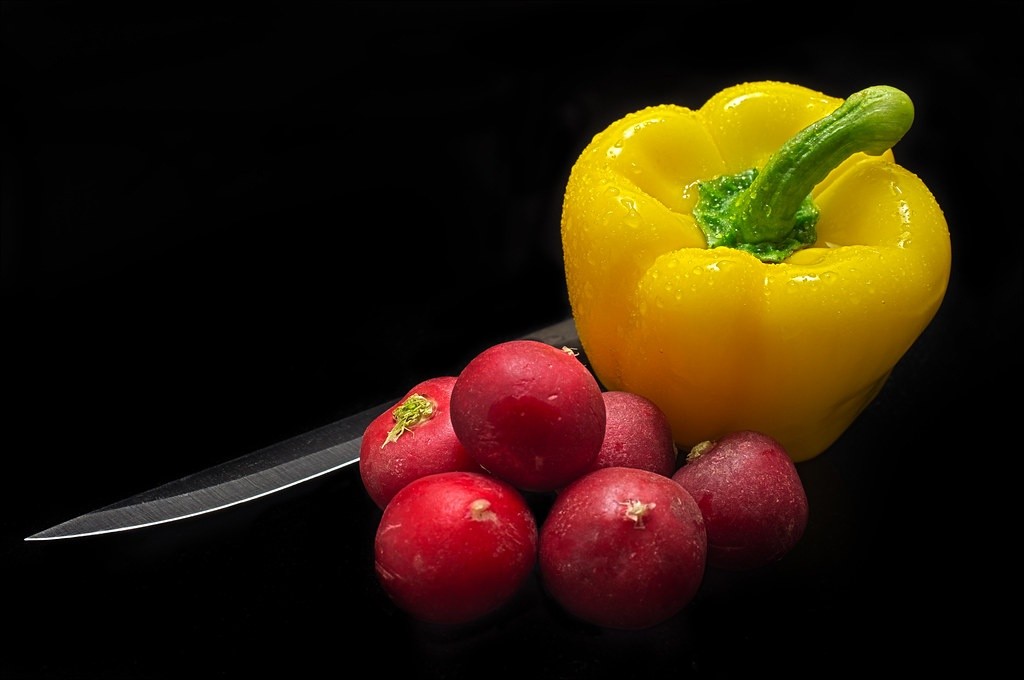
24;321;583;541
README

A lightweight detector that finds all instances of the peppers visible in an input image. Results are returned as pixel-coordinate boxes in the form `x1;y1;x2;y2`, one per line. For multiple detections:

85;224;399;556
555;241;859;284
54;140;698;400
560;81;951;459
